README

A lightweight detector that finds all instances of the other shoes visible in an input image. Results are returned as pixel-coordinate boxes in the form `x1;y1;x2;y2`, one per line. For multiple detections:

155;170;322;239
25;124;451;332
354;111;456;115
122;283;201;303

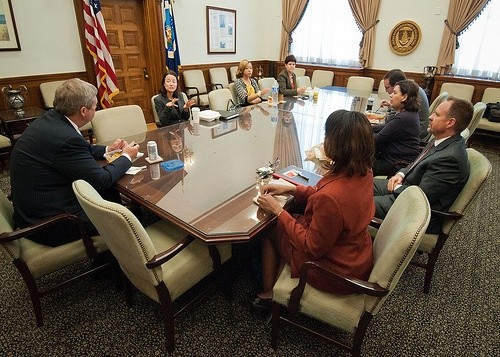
247;291;273;308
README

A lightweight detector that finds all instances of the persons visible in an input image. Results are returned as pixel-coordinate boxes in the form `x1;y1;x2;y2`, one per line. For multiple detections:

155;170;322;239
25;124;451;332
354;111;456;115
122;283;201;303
251;109;376;324
277;55;307;96
372;80;421;177
237;104;270;131
279;94;305;127
154;71;195;126
233;60;269;107
11;79;137;264
156;121;194;152
380;69;429;138
374;96;475;233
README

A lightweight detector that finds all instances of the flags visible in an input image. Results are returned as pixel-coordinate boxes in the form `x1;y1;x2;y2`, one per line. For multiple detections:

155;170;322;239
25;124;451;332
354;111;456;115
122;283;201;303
163;0;181;79
83;0;120;109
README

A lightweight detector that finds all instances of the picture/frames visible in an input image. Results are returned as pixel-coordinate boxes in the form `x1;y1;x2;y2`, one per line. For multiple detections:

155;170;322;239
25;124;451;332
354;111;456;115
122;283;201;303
0;0;22;52
206;5;236;54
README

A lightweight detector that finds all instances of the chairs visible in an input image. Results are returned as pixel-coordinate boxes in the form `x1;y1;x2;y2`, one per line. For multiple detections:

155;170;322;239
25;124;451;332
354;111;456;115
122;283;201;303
0;65;500;357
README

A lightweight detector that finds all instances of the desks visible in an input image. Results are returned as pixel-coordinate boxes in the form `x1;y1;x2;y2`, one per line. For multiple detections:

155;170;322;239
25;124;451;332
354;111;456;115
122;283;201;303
0;106;48;146
90;85;390;281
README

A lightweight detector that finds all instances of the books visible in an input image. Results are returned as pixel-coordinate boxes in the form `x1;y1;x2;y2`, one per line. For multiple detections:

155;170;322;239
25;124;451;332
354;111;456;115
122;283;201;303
216;109;240;119
272;166;323;187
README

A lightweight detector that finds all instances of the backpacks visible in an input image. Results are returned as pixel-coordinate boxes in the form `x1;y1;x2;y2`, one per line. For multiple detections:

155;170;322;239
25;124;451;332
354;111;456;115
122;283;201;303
485;101;500;123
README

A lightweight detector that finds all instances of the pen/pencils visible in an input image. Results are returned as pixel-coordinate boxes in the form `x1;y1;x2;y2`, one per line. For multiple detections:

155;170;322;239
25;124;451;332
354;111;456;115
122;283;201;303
375;106;381;113
294;171;309;181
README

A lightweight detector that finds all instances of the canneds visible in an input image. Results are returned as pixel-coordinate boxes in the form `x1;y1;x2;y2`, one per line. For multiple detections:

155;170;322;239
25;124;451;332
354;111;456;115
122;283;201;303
147;140;158;160
150;163;160;180
